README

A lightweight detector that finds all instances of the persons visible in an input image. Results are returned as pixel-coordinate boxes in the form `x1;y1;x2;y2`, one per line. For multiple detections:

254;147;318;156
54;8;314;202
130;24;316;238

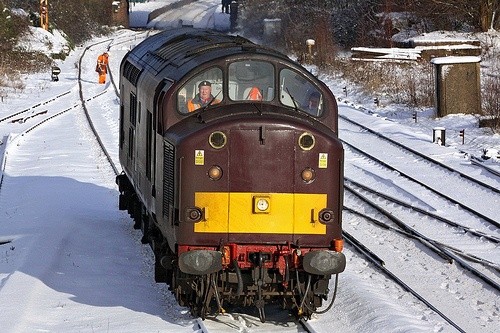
298;91;322;116
182;80;221;115
96;52;109;84
245;73;270;100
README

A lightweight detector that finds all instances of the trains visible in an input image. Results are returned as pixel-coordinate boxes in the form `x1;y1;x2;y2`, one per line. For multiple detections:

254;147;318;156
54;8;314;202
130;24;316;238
115;25;347;322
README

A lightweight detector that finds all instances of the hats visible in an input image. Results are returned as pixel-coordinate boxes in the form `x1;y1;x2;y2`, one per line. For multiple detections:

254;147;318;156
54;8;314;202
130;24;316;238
198;81;211;87
